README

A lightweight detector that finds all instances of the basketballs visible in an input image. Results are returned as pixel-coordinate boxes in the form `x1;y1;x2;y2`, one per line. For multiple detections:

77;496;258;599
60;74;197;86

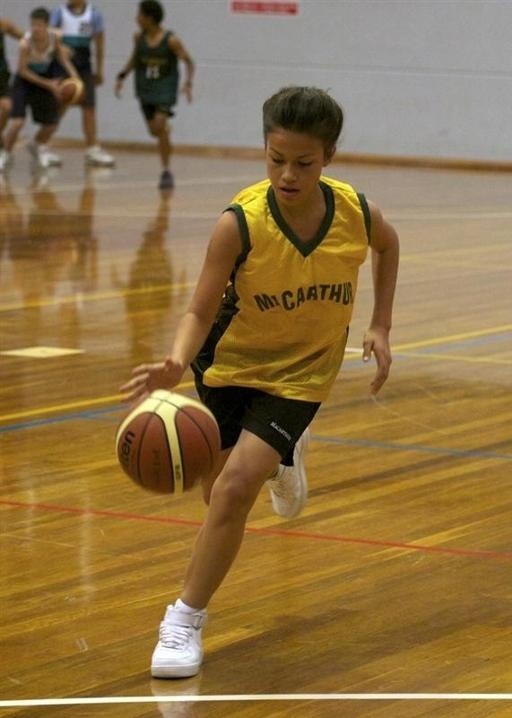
116;389;221;496
56;78;86;106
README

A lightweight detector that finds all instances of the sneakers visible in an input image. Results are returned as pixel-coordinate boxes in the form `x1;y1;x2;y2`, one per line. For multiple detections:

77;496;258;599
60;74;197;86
157;170;172;191
267;427;308;518
151;604;204;678
1;142;117;170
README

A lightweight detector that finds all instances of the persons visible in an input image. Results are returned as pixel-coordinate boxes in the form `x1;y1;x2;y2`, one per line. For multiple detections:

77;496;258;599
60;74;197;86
0;6;81;170
0;16;73;131
116;84;401;681
114;0;196;188
49;0;115;168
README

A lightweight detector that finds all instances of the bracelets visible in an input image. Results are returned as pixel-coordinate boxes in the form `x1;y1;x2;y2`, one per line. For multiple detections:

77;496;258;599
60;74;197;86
119;72;125;80
